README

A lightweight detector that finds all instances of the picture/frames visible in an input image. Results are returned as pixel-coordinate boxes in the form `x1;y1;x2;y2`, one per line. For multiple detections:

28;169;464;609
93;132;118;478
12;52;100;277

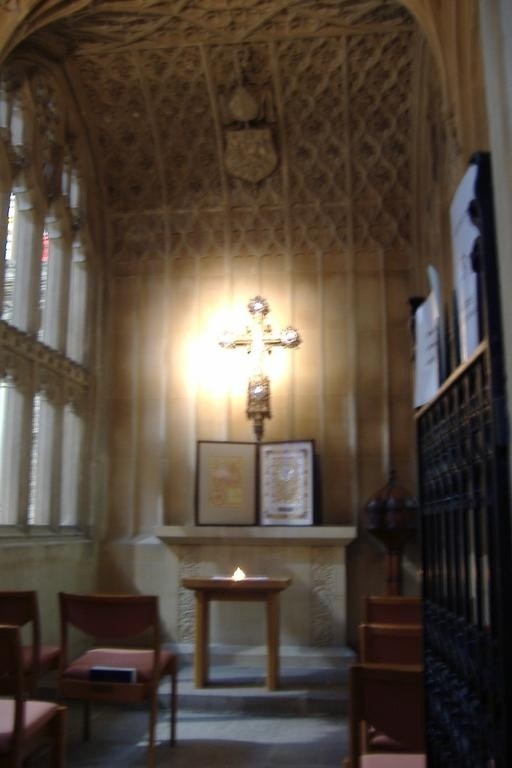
195;440;258;527
257;439;314;527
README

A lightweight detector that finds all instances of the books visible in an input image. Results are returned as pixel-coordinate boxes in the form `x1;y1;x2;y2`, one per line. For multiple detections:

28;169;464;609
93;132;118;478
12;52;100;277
89;665;138;684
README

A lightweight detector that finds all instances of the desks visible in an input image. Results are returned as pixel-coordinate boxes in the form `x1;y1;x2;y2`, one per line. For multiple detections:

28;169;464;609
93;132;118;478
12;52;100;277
182;576;291;690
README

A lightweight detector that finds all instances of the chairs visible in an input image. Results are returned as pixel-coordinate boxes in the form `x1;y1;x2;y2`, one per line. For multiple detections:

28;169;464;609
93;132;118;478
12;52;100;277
342;592;428;768
0;589;178;768
